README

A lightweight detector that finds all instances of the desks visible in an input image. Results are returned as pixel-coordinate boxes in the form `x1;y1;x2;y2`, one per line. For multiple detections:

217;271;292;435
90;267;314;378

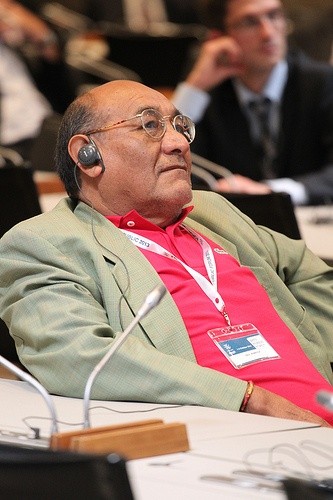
38;192;333;260
0;379;333;499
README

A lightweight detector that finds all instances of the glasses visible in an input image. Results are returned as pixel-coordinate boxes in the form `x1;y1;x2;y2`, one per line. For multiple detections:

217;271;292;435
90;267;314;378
84;108;195;144
221;8;285;30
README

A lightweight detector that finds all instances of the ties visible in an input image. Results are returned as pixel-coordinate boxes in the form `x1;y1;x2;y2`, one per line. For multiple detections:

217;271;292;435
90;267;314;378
247;97;272;163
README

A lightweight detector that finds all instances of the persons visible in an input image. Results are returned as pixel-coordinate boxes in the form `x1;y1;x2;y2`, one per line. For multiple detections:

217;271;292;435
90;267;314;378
0;80;333;430
168;0;333;207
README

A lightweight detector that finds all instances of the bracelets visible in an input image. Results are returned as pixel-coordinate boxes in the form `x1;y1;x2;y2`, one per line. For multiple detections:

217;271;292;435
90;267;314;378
239;380;255;413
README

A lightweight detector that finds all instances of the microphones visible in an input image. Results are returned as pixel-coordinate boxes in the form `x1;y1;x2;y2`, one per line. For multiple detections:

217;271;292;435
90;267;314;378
83;283;167;429
0;355;58;434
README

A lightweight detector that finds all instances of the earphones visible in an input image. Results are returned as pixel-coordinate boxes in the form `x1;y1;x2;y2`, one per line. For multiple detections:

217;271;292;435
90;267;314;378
78;144;99;167
